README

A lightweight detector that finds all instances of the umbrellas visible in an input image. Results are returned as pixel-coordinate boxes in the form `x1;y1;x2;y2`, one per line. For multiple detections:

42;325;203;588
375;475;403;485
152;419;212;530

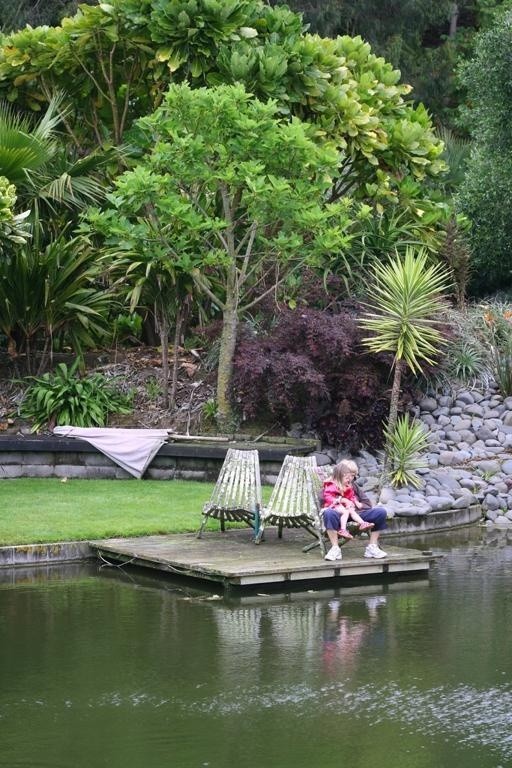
50;422;231;480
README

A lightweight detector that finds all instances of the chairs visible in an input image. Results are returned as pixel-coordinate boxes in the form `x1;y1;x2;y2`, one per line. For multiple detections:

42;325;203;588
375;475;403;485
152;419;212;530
198;449;265;542
302;463;383;556
257;455;320;538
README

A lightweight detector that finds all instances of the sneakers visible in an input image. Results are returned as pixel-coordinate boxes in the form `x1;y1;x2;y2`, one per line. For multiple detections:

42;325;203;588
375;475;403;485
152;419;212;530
359;521;375;530
364;545;387;558
337;528;353;538
325;547;342;560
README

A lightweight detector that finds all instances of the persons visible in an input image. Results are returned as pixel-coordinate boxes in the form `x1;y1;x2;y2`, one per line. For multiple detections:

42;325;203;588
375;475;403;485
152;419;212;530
320;458;389;561
320;594;387;693
322;461;376;539
336;615;369;666
